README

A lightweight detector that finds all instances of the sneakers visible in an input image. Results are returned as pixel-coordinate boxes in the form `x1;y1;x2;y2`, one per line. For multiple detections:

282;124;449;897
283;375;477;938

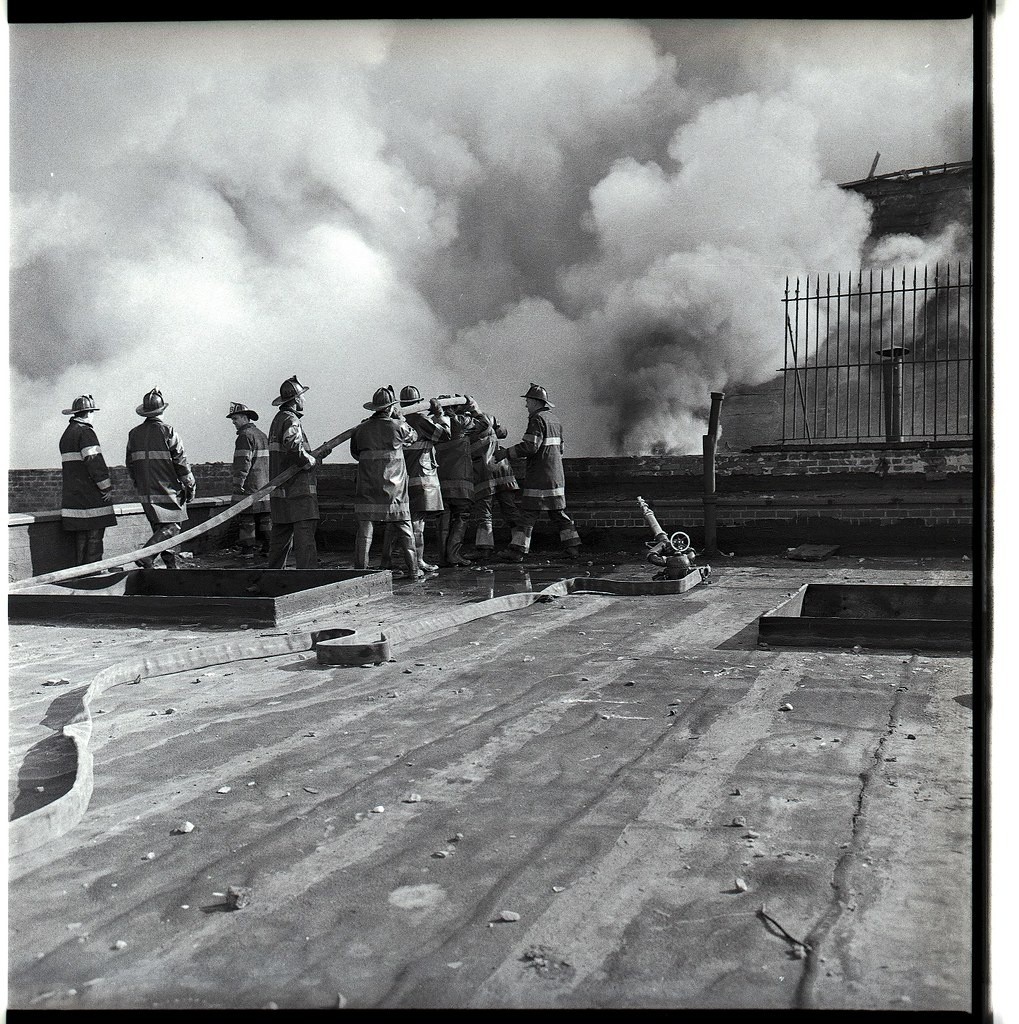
464;545;496;560
492;547;524;563
565;545;581;558
234;544;255;559
256;544;271;556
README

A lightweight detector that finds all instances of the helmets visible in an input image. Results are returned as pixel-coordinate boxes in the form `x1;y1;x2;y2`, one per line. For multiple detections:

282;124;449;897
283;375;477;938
226;402;259;421
272;374;309;406
397;385;424;403
363;384;400;411
62;395;100;415
135;385;169;416
520;383;556;408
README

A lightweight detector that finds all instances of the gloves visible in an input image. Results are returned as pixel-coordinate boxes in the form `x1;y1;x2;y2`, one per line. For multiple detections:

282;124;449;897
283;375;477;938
464;394;478;412
233;484;245;494
430;398;445;417
184;487;196;503
492;446;507;464
394;406;406;422
303;454;320;470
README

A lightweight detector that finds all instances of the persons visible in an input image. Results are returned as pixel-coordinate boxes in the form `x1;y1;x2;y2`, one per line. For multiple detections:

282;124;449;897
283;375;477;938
350;383;585;579
59;393;118;575
226;374;332;568
126;387;196;569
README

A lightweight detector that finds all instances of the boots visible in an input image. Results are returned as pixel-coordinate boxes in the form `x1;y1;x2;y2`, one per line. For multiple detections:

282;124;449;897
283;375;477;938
379;553;403;574
408;552;425;580
418;549;439;572
160;550;179;571
134;537;159;574
445;540;471;566
